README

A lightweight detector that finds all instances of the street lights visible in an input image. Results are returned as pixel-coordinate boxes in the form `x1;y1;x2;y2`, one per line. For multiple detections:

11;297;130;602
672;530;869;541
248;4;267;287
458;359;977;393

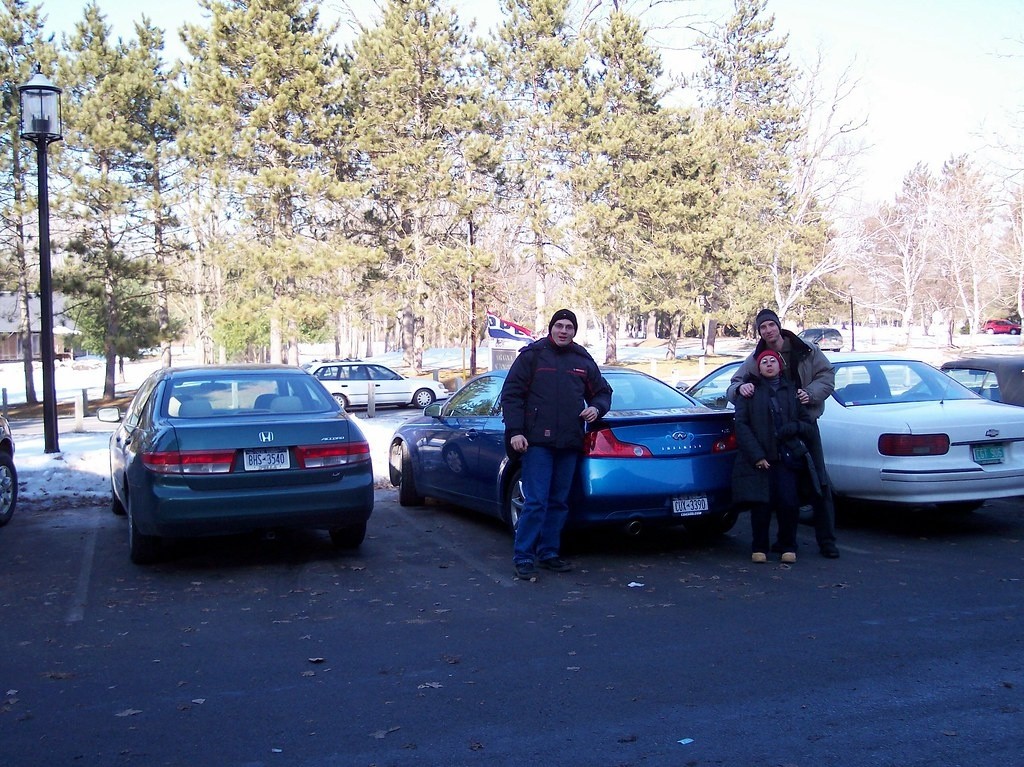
18;60;65;455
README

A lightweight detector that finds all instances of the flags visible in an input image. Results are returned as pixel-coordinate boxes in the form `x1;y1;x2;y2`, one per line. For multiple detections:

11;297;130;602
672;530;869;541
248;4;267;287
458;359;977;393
487;312;537;343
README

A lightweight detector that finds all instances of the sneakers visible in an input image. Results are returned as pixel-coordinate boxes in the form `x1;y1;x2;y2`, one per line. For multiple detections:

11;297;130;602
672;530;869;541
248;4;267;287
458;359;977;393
536;557;572;571
513;563;538;579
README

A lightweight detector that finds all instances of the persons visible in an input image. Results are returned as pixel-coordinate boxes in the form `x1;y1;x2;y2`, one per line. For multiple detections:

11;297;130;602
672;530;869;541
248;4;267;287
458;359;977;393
726;309;840;559
501;309;613;579
733;350;818;564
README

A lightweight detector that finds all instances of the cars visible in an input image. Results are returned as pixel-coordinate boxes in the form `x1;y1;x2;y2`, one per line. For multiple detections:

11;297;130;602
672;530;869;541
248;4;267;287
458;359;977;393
983;318;1022;335
796;327;844;352
290;360;451;411
95;362;376;572
0;414;19;529
389;365;749;545
675;350;1024;525
903;352;1024;408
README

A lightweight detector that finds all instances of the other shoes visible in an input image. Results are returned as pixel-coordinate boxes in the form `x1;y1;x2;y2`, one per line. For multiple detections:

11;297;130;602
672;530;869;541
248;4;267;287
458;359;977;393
821;548;839;559
781;552;797;563
752;553;766;562
773;543;781;552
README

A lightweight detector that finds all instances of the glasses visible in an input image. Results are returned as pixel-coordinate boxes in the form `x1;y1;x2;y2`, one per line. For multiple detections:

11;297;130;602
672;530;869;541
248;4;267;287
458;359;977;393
760;359;778;365
554;323;574;332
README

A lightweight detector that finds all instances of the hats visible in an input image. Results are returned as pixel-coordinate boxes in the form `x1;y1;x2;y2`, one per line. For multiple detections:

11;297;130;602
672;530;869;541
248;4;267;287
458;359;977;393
549;309;578;335
757;350;782;370
756;309;781;336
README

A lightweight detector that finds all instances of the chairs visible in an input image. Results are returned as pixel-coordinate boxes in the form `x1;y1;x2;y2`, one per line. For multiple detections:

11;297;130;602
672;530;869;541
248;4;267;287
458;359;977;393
178;400;213;416
845;383;874;401
270;395;303;413
254;394;278;410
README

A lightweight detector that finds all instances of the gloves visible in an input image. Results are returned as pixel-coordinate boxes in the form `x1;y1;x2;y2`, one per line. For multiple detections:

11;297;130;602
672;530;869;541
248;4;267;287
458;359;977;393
778;421;798;437
